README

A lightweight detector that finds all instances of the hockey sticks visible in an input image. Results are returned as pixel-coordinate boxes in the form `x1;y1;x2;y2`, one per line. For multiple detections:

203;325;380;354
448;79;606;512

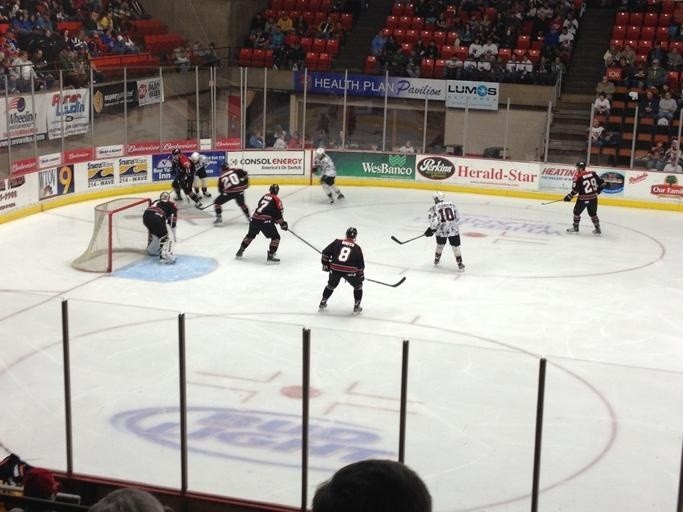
172;228;177;252
365;278;407;287
391;234;427;244
194;203;214;210
542;200;564;205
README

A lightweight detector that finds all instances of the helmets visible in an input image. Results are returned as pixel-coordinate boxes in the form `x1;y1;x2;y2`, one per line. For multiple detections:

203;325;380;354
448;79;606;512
434;192;448;203
216;162;229;172
576;161;586;168
347;227;357;239
315;147;325;159
159;192;170;200
172;148;200;161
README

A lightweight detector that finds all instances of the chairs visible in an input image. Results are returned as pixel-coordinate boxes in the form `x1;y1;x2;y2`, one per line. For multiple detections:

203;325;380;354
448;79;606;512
238;1;353;71
586;0;683;173
364;2;580;76
0;1;206;95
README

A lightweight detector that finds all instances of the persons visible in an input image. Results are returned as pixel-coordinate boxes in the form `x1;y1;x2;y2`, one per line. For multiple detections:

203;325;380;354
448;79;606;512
236;184;290;263
19;465;60;499
313;147;345;202
309;460;433;511
88;489;167;512
167;148;202;207
142;193;180;263
424;192;465;272
188;152;212;199
561;160;604;235
319;226;363;314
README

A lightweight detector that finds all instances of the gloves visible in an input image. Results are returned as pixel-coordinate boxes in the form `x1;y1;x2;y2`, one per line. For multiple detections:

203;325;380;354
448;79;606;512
280;221;288;232
356;271;365;282
320;262;333;273
424;227;437;238
564;194;573;202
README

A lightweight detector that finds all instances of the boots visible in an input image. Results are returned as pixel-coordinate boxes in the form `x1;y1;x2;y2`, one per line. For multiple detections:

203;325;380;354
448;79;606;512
213;215;223;225
319;299;327;309
432;258;465;269
592;225;601;234
354;303;363;312
567;224;579;232
267;251;280;262
236;248;245;257
173;192;211;205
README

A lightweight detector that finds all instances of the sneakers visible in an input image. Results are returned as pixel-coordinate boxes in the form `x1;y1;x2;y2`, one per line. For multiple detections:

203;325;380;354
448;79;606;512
145;249;177;265
327;192;344;204
269;183;279;193
